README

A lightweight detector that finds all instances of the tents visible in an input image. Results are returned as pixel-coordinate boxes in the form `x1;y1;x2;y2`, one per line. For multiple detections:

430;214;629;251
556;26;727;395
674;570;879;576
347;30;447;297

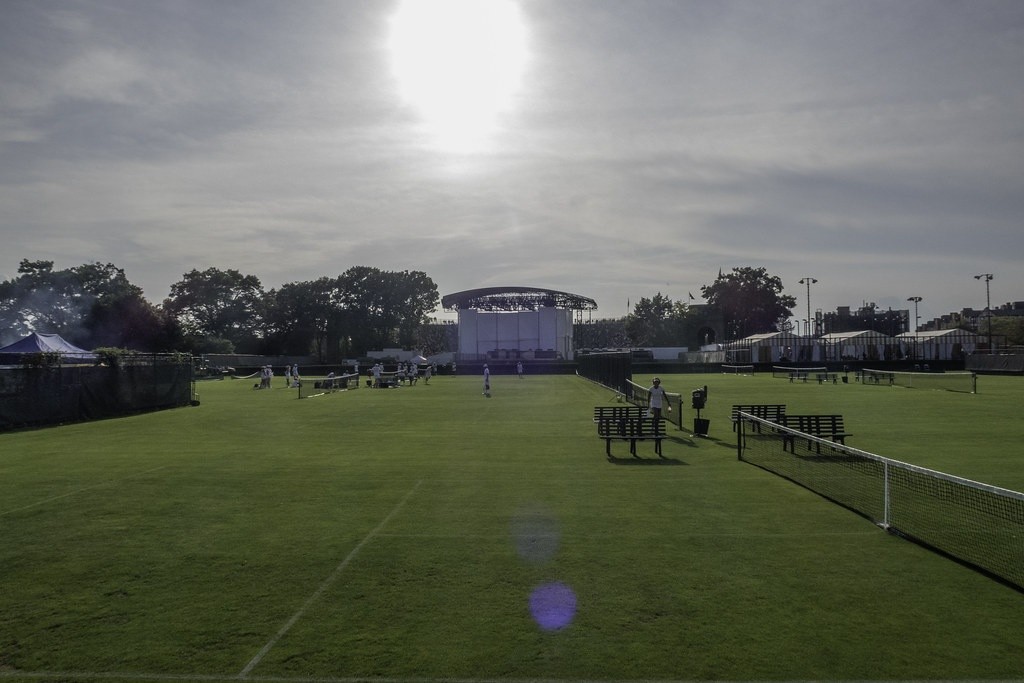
0;332;96;358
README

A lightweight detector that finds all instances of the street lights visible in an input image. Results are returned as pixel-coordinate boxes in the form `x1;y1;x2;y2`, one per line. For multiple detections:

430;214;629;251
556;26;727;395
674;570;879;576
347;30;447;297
973;273;994;354
906;296;922;362
798;277;818;368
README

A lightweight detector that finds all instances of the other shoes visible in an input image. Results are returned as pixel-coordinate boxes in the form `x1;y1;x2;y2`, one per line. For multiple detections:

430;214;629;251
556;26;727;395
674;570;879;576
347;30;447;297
287;386;290;388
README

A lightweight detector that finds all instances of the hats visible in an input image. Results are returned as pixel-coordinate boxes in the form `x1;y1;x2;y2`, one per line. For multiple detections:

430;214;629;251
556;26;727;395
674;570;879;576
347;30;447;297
261;364;272;369
653;378;660;382
295;364;297;366
483;364;488;368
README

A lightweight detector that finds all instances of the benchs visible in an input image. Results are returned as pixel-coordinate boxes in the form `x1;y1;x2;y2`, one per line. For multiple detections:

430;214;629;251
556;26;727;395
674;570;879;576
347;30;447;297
778;414;852;454
815;373;839;385
727;403;786;432
854;371;896;384
592;406;647;424
597;418;668;457
788;372;810;383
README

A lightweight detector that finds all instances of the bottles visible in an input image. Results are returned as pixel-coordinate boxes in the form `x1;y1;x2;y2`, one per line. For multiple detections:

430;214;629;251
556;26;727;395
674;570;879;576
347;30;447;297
668;406;672;414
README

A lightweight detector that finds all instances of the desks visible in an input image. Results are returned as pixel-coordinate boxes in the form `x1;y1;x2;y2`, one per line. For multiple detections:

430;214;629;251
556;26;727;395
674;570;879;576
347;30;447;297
487;349;528;359
534;350;557;359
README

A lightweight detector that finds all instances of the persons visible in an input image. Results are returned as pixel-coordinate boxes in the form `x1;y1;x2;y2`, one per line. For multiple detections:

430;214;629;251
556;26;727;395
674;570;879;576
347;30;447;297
647;377;671;429
343;370;349;376
371;363;384;388
315;372;334;389
398;361;437;386
285;364;300;388
517;362;523;378
451;361;457;378
260;364;273;389
482;363;490;395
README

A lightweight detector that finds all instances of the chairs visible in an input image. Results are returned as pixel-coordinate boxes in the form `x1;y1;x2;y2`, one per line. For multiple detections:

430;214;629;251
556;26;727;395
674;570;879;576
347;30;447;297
915;364;920;371
924;364;930;371
